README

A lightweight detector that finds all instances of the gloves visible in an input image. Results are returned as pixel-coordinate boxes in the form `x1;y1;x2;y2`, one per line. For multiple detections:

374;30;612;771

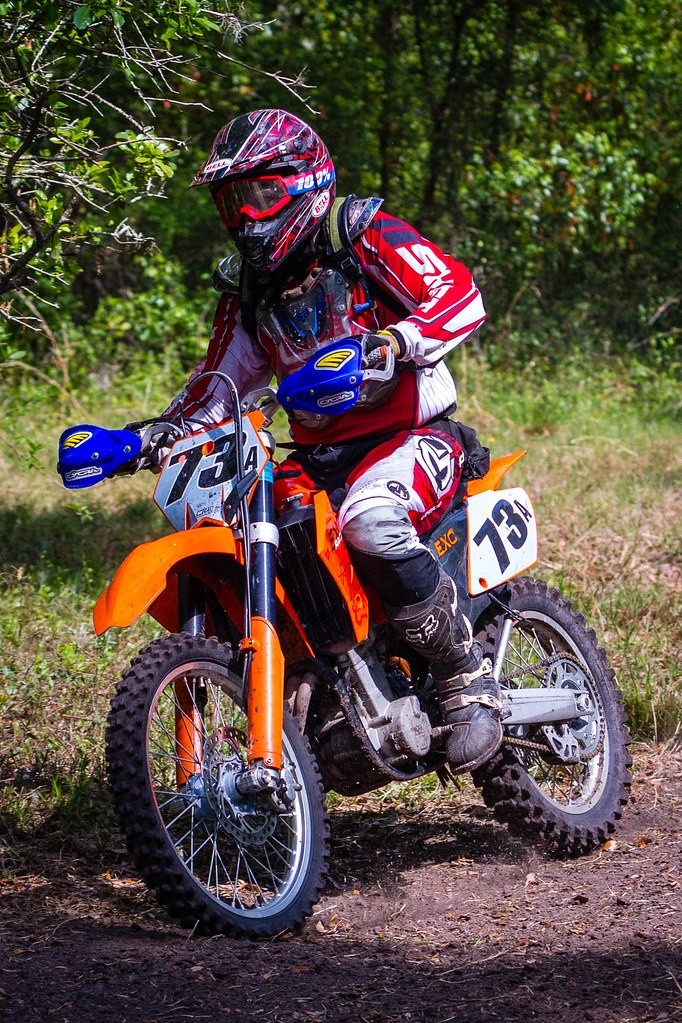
362;334;390;367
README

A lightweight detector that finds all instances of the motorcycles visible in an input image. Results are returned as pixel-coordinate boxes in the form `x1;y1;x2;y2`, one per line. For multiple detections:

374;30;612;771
57;338;634;942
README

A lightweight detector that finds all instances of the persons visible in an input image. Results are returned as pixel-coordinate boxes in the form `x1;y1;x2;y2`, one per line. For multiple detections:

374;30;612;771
111;109;503;775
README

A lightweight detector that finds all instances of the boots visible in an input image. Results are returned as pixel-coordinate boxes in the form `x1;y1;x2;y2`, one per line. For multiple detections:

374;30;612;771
383;566;502;775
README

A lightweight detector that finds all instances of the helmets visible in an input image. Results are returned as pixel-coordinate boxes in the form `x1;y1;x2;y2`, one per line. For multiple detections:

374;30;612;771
188;108;335;281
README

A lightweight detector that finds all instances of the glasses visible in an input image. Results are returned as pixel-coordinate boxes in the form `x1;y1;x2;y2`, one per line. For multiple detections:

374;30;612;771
215;174;291;230
